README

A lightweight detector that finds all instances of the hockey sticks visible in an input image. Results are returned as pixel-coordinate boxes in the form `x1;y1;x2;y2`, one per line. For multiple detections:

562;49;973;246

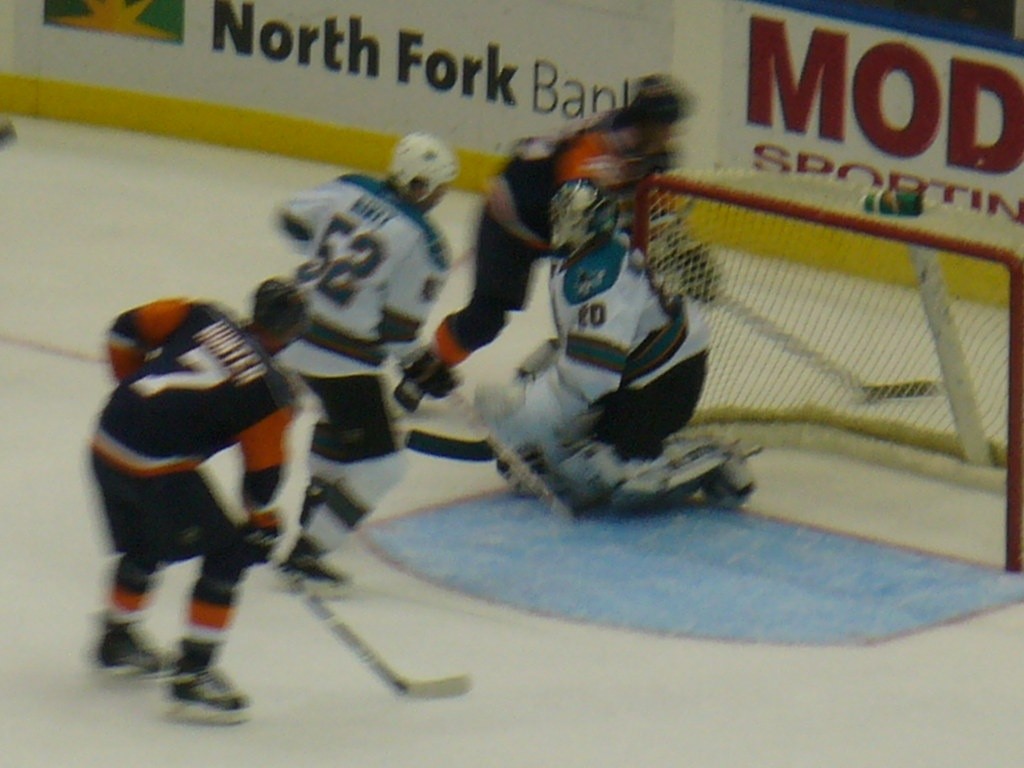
198;462;476;702
691;280;941;406
405;343;578;525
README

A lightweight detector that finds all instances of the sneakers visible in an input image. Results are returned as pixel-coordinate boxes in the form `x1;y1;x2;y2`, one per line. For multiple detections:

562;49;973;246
154;637;254;724
277;534;346;591
394;376;427;412
97;622;160;673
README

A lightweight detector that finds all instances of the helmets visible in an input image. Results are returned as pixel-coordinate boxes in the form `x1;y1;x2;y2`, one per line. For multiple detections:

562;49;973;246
550;178;619;260
393;133;459;203
254;279;311;339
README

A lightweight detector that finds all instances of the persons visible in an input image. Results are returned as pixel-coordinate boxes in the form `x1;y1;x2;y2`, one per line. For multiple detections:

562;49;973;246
476;179;754;509
270;132;460;597
390;75;689;418
92;276;314;723
